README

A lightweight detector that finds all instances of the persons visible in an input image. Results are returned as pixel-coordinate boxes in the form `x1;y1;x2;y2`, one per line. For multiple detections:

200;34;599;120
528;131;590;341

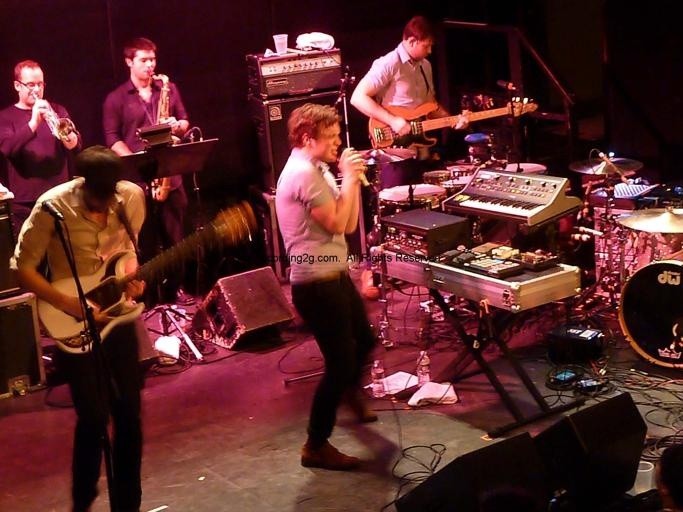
654;443;683;512
276;102;379;470
9;145;147;512
0;60;83;336
350;15;473;289
103;37;197;306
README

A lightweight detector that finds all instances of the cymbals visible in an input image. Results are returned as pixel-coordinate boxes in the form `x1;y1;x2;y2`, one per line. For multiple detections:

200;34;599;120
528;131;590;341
337;148;416;165
568;157;643;175
615;207;683;235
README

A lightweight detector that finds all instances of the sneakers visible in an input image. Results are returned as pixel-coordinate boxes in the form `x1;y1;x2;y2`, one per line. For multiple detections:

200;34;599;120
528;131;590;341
342;390;377;422
301;442;358;473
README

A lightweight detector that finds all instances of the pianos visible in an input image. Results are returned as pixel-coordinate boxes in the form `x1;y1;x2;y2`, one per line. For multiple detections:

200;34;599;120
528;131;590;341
439;167;579;227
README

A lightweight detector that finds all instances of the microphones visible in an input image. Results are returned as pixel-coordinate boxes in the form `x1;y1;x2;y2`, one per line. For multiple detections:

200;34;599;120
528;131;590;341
344;149;371;186
41;199;65;221
599;152;631;185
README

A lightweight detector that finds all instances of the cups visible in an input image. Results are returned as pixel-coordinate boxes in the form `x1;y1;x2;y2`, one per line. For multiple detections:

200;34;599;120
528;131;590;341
272;34;288;54
633;461;655;496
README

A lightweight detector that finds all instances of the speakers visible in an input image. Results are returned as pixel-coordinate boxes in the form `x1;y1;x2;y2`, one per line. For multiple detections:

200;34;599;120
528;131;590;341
192;265;296;351
395;432;559;512
533;391;648;502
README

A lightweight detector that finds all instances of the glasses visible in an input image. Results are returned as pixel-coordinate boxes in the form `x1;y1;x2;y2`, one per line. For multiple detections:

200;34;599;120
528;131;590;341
16;79;45;90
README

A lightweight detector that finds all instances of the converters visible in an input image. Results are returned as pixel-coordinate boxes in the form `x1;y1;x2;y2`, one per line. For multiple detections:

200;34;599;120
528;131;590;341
576;376;608;389
550;369;577;386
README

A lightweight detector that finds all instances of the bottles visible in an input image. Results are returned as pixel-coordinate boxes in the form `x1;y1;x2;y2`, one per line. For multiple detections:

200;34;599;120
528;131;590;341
370;360;386;398
460;92;471;115
416;350;431;385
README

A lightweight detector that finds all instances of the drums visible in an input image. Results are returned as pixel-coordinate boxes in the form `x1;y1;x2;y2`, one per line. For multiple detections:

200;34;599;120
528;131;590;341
443;174;472;198
377;183;446;227
423;170;450;184
618;259;682;368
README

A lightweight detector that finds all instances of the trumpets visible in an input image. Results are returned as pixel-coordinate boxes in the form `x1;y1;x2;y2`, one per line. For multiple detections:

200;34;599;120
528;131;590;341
32;92;71;140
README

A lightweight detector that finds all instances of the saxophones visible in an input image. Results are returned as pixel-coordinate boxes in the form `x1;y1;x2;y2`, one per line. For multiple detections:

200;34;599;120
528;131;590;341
147;71;178;201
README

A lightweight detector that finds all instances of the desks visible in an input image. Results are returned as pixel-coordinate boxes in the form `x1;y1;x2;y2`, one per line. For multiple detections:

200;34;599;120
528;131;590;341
396;283;588;441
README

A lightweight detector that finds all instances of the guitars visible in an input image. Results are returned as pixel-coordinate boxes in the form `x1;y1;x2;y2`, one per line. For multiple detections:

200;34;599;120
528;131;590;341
368;97;538;149
34;200;255;354
